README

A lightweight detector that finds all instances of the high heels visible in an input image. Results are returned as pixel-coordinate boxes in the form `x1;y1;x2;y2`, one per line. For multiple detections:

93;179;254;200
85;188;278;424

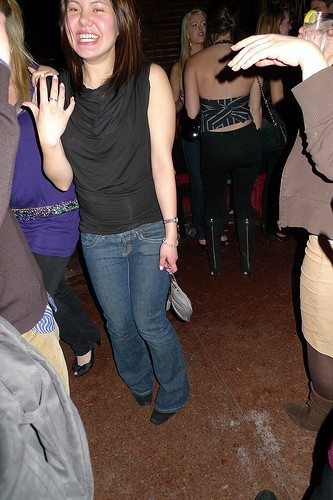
73;336;101;377
261;224;286;245
221;239;230;246
198;240;207;250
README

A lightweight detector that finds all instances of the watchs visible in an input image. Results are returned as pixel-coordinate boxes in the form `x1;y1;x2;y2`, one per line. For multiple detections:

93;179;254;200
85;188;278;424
163;217;178;224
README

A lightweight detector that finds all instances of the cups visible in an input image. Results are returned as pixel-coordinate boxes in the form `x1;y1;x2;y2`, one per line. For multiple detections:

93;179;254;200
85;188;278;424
303;12;330;54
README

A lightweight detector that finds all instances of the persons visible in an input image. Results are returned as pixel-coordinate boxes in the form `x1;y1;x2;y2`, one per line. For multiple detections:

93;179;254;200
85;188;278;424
26;1;192;427
170;9;231;251
308;0;333;21
184;1;264;278
254;5;304;244
227;12;333;437
1;58;73;398
1;0;103;379
252;439;333;500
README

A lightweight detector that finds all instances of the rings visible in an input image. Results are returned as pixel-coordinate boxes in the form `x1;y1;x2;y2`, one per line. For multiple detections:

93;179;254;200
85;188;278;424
48;97;58;102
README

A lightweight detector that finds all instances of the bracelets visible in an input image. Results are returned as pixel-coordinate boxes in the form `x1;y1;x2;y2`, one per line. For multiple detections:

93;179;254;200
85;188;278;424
162;236;179;247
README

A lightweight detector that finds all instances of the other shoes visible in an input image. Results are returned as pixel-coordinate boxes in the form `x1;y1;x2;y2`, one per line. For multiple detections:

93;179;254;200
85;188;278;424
255;490;276;500
151;408;176;425
134;393;152;406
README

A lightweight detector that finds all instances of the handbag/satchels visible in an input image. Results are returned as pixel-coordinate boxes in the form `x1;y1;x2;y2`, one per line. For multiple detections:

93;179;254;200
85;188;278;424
166;264;193;322
179;105;202;142
262;98;289;161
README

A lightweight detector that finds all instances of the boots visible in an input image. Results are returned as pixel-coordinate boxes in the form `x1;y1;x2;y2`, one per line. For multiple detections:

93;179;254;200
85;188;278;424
202;216;222;280
285;381;332;432
235;216;256;283
